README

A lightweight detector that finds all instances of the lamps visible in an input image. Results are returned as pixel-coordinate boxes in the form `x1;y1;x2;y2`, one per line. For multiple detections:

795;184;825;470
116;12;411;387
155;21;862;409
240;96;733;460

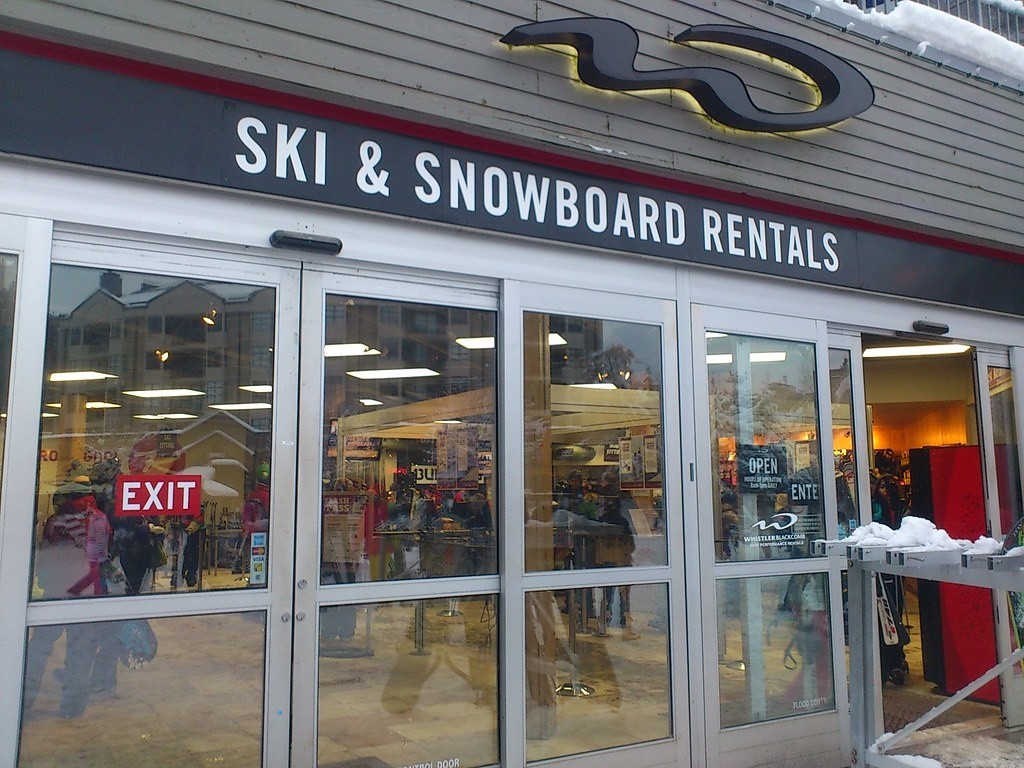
616;368;630;381
154;350;169;362
598;368;608;380
844;431;851;437
201;309;216;326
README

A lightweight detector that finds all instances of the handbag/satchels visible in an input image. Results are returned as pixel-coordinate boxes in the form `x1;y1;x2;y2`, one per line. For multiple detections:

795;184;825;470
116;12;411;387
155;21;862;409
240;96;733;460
102;566;160;671
876;573;911;650
131;533;168;569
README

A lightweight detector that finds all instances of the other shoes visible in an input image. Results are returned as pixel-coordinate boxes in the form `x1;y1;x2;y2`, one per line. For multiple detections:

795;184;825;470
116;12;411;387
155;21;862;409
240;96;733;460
54;667;65;679
562;605;568;614
86;681;110;700
607;616;625;627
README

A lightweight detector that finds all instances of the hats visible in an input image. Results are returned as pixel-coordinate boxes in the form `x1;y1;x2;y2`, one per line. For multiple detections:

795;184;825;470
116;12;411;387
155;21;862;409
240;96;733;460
91;452;123;484
64;459;92;484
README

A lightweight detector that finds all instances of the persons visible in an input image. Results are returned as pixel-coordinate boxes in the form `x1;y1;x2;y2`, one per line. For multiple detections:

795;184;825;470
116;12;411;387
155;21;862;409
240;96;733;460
22;459;207;720
551;464;639;628
866;471;910;691
